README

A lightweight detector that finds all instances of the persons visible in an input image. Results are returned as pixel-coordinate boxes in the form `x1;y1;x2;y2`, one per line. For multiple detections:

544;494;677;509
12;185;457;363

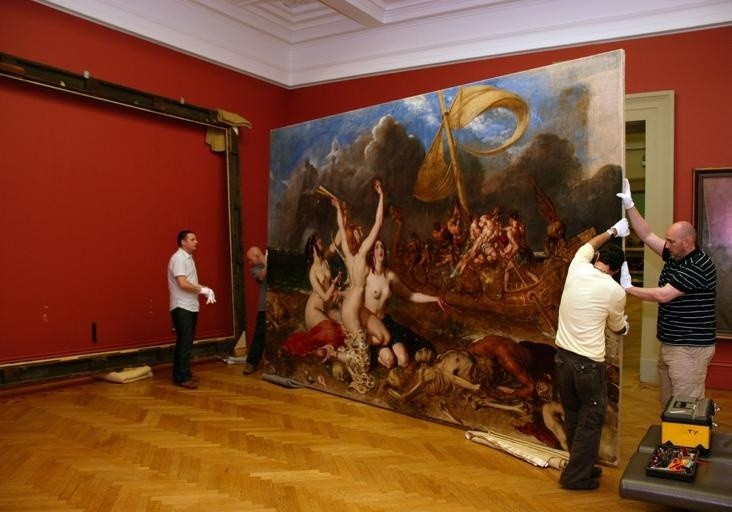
537;373;618;461
617;178;717;408
555;217;630;490
167;230;217;390
244;247;269;374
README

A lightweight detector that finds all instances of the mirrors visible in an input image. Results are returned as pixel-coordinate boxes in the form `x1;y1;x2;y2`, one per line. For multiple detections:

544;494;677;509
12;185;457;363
691;165;732;341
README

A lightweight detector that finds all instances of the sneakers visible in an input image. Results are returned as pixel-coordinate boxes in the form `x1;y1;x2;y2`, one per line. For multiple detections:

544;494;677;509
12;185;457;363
242;362;255;375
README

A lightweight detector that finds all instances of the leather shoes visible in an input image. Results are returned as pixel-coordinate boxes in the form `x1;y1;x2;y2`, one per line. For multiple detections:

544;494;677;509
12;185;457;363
591;466;602;479
178;380;199;390
583;478;600;490
191;375;200;382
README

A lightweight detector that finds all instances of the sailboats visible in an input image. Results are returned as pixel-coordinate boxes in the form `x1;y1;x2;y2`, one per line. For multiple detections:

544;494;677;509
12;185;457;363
390;85;592;319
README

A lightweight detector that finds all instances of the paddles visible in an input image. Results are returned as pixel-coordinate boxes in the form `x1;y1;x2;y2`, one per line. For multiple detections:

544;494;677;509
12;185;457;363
496;240;556;339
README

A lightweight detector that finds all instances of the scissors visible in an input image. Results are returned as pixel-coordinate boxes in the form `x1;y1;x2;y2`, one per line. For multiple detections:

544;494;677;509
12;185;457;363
665;458;686;471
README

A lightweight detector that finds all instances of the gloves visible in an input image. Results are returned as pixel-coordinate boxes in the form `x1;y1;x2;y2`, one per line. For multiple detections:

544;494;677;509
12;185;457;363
621;313;631;337
609;217;631;237
615;177;636;213
619;260;634;290
199;286;217;305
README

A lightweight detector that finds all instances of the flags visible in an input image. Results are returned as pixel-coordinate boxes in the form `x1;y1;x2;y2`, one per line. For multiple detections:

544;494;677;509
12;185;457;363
414;85;530;203
